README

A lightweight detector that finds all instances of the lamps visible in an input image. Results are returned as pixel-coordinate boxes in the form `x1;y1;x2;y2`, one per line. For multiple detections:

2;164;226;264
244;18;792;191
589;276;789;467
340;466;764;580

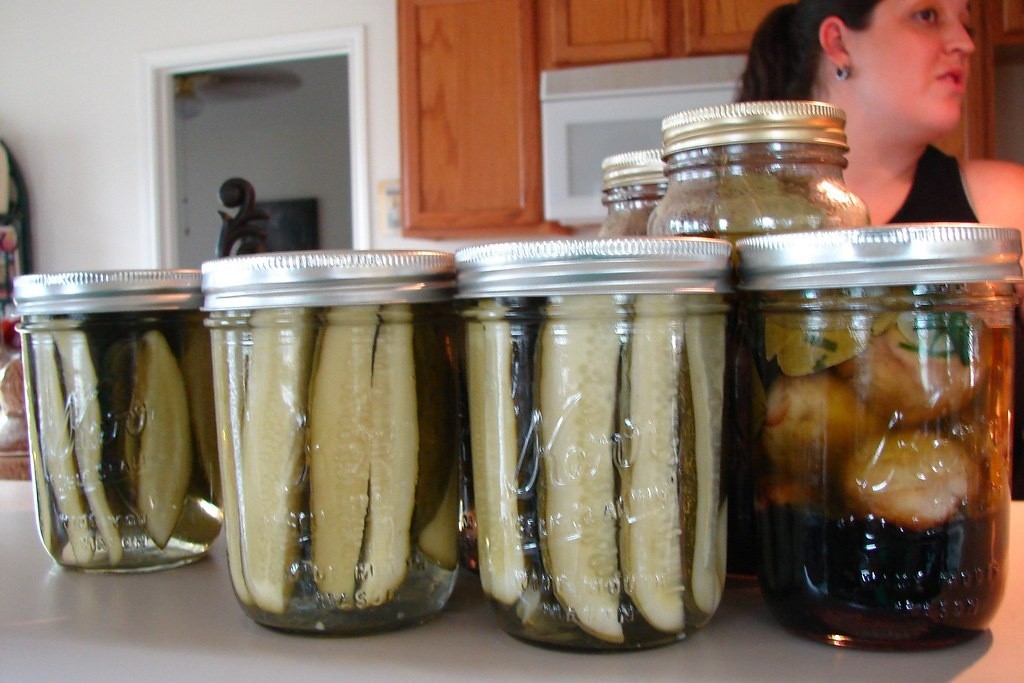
173;63;303;120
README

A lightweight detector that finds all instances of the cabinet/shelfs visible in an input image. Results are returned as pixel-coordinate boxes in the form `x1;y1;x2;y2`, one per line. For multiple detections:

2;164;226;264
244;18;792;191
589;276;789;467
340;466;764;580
395;0;1024;237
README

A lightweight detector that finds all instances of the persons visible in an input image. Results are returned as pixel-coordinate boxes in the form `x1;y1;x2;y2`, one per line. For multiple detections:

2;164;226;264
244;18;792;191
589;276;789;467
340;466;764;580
736;0;1024;501
